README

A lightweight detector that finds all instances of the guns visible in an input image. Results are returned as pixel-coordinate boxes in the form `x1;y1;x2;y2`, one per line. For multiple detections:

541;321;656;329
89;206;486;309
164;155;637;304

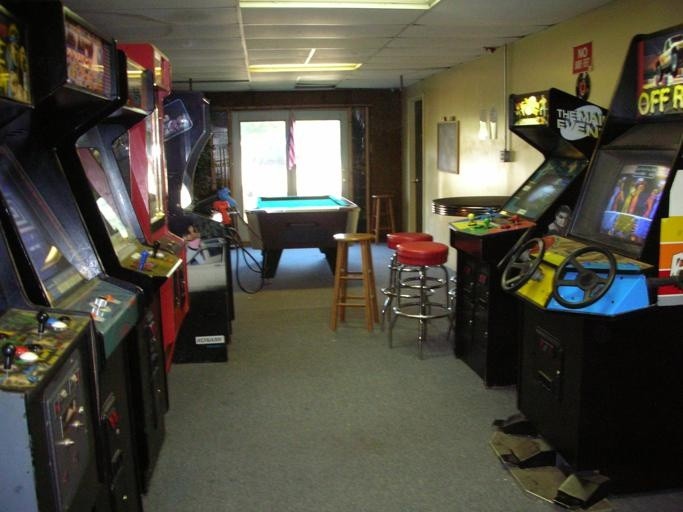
211;188;236;208
208;200;237;226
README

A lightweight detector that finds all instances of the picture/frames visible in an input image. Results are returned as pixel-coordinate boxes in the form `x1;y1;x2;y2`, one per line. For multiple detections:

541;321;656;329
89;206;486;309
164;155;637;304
435;121;460;174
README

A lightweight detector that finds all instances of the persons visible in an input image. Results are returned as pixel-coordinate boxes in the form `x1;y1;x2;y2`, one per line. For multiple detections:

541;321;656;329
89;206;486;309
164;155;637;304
548;205;572;235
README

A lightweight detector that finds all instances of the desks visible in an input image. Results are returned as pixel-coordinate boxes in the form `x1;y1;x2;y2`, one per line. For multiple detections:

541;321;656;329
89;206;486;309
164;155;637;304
247;196;359;275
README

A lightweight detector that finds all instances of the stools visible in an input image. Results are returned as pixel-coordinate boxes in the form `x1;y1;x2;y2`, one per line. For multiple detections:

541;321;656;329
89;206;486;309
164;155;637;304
370;192;397;240
333;230;451;349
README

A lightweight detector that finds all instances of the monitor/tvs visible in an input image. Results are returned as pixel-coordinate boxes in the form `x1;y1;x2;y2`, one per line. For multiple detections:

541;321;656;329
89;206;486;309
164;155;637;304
519;176;570;211
598;164;671;243
76;147;126;235
0;164;72;281
